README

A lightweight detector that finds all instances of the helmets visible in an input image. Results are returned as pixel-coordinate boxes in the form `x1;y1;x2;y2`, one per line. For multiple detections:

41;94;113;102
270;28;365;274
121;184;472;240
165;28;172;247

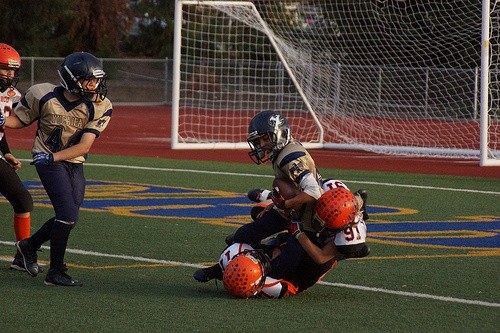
0;42;22;70
246;109;291;161
223;251;266;298
313;186;359;229
57;52;106;96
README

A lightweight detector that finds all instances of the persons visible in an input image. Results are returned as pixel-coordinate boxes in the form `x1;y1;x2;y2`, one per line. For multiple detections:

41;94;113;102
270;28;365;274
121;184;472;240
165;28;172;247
194;109;371;299
0;51;114;286
0;43;47;274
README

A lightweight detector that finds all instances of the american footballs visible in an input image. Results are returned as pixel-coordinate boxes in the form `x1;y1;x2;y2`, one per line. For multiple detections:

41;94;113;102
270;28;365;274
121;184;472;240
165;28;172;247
271;176;304;219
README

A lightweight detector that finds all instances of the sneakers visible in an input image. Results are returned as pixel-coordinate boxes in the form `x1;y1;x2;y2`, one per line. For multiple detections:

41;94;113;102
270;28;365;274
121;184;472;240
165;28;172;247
11;255;44;273
16;237;40;278
192;262;224;283
355;189;369;221
44;268;85;287
247;188;273;202
225;233;236;245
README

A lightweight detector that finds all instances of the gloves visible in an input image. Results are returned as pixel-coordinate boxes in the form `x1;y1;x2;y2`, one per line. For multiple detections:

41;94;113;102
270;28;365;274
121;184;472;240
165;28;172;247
270;188;290;211
0;114;5;126
285;207;299;220
287;215;305;239
33;152;54;166
